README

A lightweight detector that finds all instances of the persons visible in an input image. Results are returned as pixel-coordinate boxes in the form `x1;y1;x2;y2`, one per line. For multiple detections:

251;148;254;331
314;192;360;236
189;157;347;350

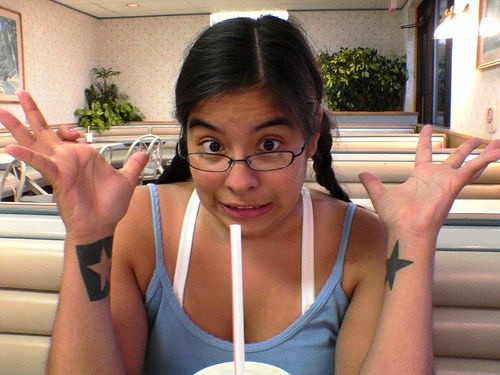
0;14;500;374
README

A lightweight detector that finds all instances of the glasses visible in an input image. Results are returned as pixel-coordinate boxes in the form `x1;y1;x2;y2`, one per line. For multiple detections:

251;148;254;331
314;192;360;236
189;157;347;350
175;127;308;172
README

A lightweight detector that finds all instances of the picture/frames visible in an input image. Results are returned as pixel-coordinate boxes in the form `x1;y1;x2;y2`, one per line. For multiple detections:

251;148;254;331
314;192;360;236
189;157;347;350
0;6;25;104
476;0;500;70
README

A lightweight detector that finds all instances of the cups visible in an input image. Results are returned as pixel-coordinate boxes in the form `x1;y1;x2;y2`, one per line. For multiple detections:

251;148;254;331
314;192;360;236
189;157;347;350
192;361;291;375
87;133;93;142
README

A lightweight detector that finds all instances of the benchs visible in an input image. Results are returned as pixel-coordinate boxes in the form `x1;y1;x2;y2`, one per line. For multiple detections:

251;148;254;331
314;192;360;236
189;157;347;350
0;121;500;375
